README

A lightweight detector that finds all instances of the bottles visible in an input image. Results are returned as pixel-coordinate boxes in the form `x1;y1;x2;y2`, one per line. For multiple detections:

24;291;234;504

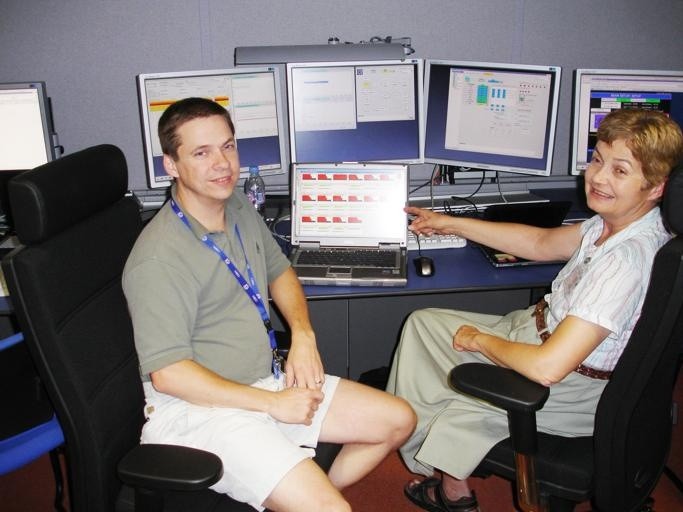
243;164;266;221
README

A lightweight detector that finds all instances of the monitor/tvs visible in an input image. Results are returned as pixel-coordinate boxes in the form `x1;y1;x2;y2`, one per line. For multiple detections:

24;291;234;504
0;81;56;170
135;67;288;190
284;58;425;165
423;58;564;177
567;68;683;176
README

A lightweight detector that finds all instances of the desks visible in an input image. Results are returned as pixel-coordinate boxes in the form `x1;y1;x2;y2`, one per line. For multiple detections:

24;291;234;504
0;215;585;389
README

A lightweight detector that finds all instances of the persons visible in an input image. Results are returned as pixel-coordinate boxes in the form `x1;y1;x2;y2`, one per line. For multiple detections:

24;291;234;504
386;110;683;512
122;97;417;512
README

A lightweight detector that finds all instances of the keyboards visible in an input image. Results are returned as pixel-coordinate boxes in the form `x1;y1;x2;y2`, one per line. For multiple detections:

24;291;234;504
407;229;467;251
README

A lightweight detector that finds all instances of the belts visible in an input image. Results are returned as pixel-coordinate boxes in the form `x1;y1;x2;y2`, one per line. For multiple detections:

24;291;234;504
535;300;613;379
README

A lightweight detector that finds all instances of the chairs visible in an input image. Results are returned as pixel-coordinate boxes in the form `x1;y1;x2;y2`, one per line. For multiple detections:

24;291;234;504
0;332;67;512
450;234;683;512
2;144;342;512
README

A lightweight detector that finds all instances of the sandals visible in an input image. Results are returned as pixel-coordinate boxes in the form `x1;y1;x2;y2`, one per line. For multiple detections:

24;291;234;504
470;471;492;479
405;477;480;512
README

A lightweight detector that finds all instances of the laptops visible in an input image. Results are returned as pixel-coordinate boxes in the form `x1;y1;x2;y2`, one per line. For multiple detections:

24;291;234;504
477;199;574;269
288;161;411;287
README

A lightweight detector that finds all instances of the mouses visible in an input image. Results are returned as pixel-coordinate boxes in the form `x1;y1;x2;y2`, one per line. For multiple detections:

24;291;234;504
413;256;435;278
